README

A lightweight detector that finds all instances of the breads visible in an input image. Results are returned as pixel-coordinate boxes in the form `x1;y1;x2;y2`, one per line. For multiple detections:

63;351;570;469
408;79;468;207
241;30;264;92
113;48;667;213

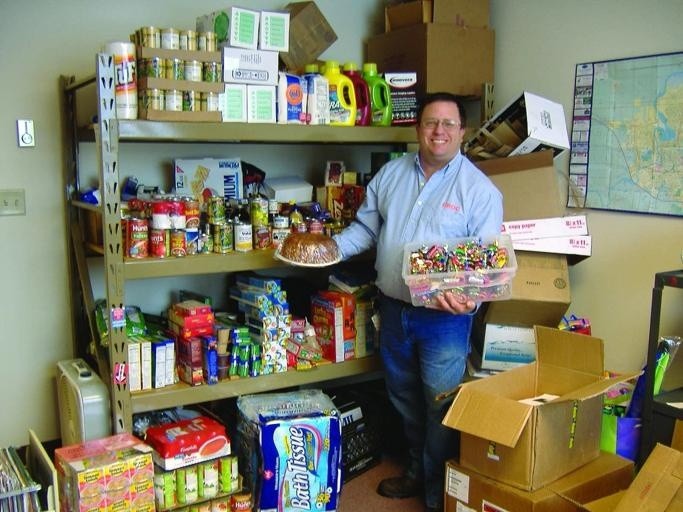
280;232;338;265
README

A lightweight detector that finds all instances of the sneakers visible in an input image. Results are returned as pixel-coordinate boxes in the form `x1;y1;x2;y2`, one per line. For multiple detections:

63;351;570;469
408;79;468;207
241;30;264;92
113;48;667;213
377;477;422;498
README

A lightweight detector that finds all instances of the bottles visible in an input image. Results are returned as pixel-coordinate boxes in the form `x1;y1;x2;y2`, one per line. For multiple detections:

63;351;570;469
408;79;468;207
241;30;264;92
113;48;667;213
289;202;303;223
303;63;318;75
319;61;325;75
223;202;251;224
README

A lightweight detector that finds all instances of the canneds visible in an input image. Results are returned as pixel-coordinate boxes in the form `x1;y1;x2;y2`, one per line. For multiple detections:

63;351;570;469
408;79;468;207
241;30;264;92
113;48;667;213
130;27;223;112
153;454;253;512
119;197;271;261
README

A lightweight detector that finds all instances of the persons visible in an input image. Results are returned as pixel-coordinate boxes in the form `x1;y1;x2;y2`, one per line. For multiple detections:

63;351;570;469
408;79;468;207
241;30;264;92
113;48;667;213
329;91;505;512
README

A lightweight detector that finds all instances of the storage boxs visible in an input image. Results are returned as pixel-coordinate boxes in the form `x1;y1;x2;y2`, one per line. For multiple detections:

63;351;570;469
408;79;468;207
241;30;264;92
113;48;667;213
444;268;683;512
195;1;337;123
367;0;495;98
460;92;592;329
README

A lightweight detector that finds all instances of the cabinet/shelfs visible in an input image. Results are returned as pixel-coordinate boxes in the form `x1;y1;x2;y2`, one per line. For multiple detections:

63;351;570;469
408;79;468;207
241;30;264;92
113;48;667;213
61;73;384;416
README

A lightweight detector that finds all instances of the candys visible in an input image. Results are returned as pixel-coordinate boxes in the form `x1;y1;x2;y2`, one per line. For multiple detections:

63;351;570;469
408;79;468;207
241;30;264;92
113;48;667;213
406;238;513;305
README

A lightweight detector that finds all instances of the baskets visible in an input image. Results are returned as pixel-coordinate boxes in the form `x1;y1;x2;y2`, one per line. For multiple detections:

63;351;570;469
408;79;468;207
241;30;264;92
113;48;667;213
343;410;382;483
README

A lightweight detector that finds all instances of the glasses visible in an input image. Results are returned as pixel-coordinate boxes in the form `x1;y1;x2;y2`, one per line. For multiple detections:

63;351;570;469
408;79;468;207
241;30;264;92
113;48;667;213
422;117;458;129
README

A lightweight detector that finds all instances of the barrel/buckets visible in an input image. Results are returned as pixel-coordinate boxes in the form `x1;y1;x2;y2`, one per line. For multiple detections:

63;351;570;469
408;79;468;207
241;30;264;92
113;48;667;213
323;59;393;127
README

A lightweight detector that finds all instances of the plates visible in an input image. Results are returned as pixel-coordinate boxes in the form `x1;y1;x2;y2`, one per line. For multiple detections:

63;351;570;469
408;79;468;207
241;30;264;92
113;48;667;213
272;244;343;269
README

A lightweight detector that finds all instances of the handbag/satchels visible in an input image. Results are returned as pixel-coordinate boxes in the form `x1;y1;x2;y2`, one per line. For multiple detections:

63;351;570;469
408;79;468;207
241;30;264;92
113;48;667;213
600;414;644;462
